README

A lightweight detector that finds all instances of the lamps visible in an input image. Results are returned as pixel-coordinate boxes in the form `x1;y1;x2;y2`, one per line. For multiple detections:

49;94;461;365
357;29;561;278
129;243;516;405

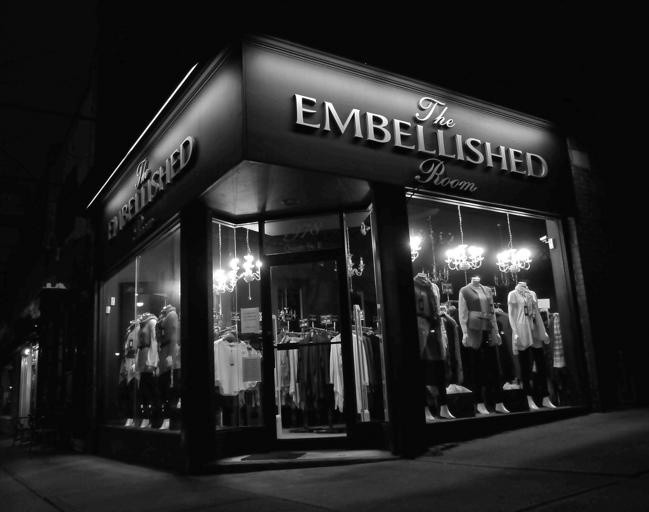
213;224;262;315
411;205;532;274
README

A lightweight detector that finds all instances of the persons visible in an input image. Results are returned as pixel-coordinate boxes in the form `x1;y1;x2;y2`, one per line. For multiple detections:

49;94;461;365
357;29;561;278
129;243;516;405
458;276;510;415
117;319;140;418
135;312;158;417
154;305;181;417
506;281;558;411
413;272;456;421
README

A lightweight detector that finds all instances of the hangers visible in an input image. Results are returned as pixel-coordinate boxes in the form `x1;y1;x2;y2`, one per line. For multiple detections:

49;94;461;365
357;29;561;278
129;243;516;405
221;328;241;344
